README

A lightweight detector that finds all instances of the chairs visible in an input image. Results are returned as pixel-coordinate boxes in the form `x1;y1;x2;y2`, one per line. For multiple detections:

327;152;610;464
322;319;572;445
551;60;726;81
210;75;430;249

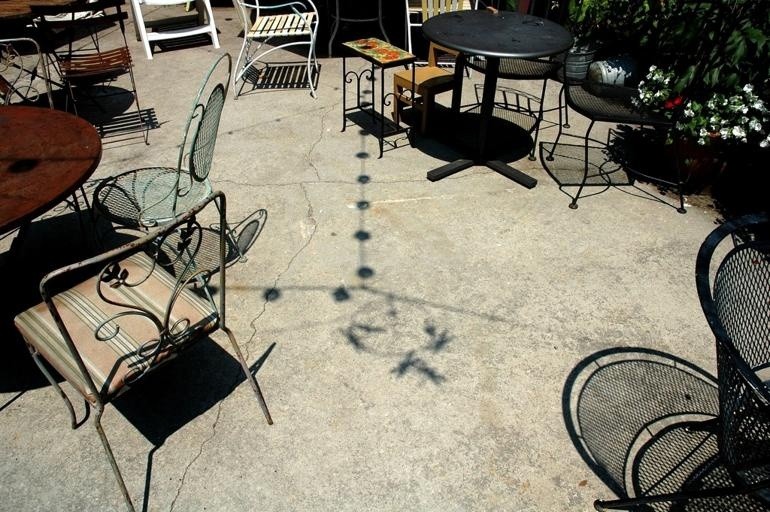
403;2;473;78
131;0;221;60
93;54;246;313
461;1;571;160
328;1;392;57
12;191;273;511
546;33;710;213
29;1;149;146
594;209;768;511
232;1;319;98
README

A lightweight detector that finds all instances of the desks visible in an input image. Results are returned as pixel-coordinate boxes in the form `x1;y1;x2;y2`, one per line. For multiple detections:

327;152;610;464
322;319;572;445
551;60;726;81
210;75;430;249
1;109;101;287
0;1;63;110
422;11;575;189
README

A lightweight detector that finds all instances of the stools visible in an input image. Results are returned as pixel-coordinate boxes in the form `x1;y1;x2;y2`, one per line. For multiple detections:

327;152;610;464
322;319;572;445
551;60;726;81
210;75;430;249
393;45;461;138
342;38;416;159
